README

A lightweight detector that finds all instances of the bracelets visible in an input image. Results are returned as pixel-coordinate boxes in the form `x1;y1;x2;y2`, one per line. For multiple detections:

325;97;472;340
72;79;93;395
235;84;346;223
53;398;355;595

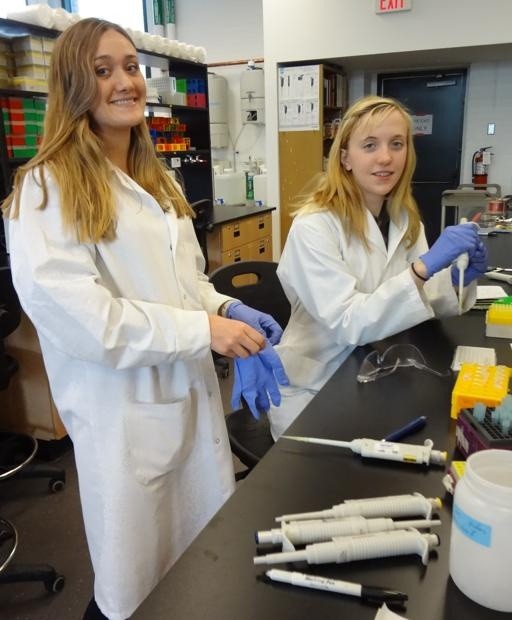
411;261;430;284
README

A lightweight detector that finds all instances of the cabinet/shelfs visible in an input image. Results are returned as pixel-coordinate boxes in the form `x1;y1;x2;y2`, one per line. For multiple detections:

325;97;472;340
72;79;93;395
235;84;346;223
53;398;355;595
206;212;277;288
277;65;349;254
1;18;215;236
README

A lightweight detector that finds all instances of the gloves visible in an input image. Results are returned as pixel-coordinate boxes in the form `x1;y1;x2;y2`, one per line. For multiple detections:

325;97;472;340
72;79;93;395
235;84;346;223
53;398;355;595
450;241;487;288
224;302;284;346
230;339;290;421
418;222;481;278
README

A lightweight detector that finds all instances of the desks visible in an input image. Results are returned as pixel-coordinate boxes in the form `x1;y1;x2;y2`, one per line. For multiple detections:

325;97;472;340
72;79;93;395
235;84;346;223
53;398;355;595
126;232;511;620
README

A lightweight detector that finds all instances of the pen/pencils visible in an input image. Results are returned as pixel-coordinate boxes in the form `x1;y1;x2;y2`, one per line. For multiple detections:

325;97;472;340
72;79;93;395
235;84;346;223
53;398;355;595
257;569;409;605
381;413;428;442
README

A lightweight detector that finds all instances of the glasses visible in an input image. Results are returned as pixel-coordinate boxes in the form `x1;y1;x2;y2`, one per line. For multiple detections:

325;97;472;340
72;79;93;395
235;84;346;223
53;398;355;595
356;343;452;385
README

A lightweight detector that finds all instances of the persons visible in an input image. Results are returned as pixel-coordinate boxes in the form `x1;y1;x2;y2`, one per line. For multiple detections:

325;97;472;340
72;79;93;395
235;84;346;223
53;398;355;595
1;15;282;620
264;93;491;445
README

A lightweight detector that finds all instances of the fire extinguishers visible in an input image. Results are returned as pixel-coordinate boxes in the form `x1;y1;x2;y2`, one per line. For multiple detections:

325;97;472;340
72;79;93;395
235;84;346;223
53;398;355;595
471;147;495;191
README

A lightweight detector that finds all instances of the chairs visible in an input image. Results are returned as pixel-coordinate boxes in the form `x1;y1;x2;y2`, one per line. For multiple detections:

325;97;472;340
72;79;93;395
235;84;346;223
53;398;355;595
206;261;291;482
0;265;66;593
